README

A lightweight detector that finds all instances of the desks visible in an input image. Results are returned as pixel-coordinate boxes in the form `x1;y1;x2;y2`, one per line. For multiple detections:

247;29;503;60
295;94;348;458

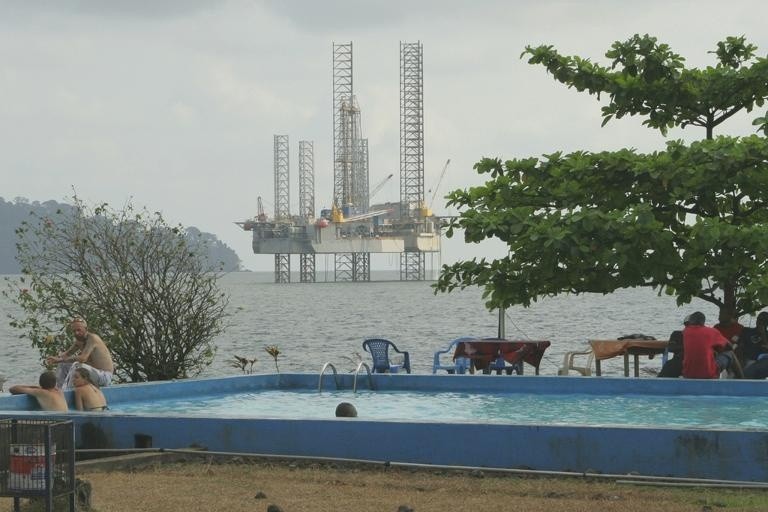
453;339;551;376
588;338;674;377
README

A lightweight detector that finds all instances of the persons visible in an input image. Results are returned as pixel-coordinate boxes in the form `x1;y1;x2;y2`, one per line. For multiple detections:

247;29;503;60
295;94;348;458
46;316;113;389
72;366;108;412
333;401;360;418
656;305;768;378
10;372;69;413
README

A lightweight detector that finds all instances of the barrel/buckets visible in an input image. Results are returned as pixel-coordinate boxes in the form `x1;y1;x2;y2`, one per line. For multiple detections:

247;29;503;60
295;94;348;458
10;443;56;492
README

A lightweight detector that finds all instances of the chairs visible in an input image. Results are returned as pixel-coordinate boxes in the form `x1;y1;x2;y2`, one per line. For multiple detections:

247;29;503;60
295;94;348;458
558;345;596;376
481;337;524;376
362;337;411;375
431;337;476;376
